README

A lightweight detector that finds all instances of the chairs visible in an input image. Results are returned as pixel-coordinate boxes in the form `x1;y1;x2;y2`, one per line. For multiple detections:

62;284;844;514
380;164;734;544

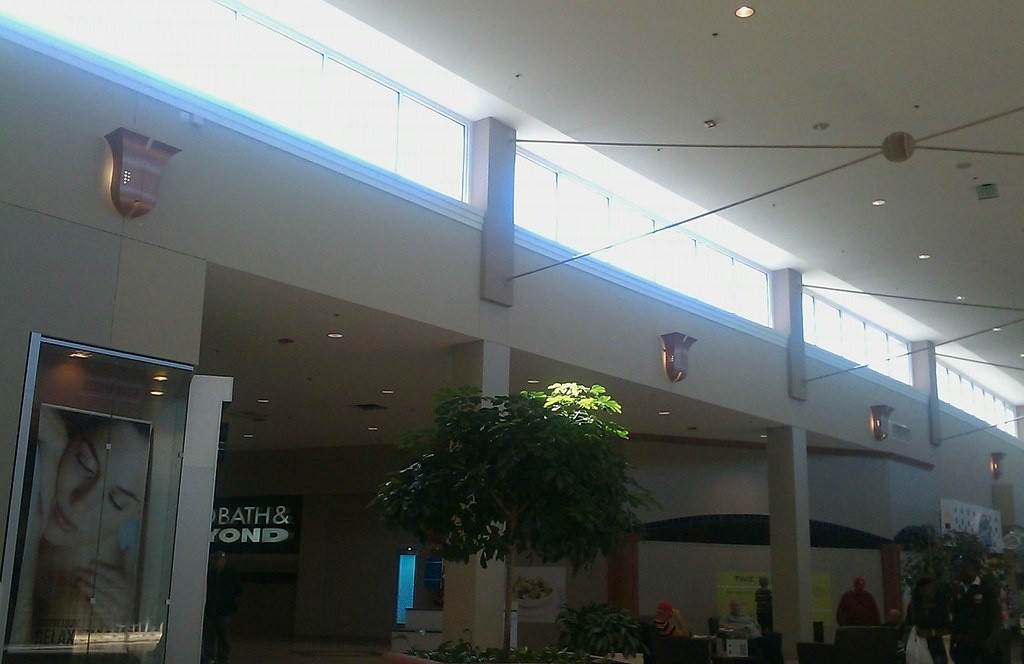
635;613;703;664
796;626;883;664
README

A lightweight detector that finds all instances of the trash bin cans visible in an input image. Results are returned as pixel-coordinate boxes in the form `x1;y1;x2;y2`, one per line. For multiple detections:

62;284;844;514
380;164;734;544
813;621;824;641
988;630;1012;664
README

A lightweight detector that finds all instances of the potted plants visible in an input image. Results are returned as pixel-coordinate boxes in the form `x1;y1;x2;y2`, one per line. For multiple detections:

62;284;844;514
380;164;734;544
376;381;652;664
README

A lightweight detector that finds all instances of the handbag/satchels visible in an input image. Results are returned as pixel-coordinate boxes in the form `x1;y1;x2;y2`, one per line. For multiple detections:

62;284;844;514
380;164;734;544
906;626;934;664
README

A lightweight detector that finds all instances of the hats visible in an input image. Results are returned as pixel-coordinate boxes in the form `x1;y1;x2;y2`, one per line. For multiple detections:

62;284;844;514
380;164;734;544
655;602;672;621
855;578;866;588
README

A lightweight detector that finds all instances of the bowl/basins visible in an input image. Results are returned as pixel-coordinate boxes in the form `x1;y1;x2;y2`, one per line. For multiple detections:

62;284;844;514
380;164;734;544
516;587;556;608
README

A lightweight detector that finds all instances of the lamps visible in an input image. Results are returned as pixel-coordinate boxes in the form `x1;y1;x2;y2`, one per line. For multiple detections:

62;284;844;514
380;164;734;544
991;452;1007;477
661;332;698;382
870;405;895;441
104;127;183;217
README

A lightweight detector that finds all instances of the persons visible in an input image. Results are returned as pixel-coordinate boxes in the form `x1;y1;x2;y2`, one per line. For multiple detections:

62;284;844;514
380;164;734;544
903;577;953;664
881;610;902;626
201;549;248;664
654;599;690;638
717;598;759;639
836;578;881;626
950;584;994;664
7;404;150;646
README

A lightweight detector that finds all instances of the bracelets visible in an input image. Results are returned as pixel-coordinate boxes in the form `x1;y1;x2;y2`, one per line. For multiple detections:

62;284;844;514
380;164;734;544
931;629;935;636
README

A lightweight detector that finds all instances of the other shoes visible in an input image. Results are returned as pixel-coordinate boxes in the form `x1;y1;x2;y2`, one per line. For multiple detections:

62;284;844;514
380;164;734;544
201;657;215;664
